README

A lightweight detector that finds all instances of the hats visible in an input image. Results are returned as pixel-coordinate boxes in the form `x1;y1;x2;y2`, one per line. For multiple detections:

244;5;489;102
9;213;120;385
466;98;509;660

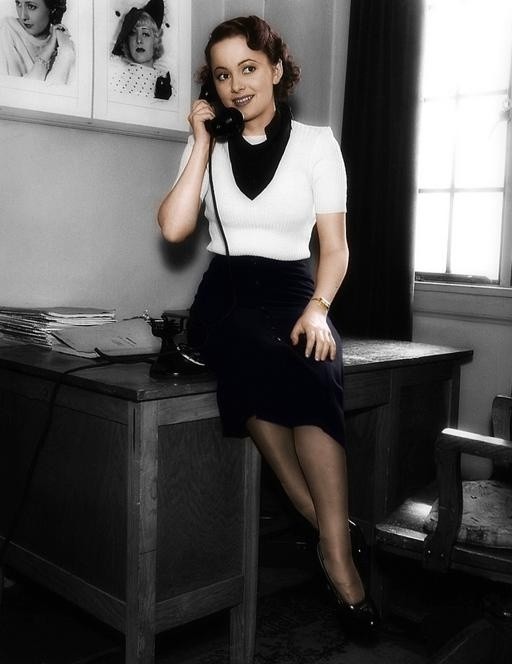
112;0;163;56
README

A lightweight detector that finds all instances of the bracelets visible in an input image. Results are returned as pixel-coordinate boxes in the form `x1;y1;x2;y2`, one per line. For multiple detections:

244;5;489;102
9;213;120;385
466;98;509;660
311;296;331;309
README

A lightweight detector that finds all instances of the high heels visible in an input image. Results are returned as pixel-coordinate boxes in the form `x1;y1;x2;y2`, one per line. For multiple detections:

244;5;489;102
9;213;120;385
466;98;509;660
317;538;377;623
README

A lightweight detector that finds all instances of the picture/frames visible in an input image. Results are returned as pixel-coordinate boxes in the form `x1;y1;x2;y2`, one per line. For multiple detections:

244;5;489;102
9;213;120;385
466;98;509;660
1;0;203;144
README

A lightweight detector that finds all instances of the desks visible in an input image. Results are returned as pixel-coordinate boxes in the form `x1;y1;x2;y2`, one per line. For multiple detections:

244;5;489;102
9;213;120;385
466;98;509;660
1;339;473;664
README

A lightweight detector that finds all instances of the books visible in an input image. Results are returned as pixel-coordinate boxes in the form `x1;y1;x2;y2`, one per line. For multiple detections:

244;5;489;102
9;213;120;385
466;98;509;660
52;317;164;358
1;304;119;353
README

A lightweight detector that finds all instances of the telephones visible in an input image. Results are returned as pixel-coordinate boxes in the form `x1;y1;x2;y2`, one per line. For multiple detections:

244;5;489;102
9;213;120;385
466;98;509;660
199;82;245;137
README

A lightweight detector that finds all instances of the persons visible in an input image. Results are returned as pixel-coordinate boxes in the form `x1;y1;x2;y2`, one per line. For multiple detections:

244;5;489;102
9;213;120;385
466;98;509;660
153;11;385;648
2;0;80;87
105;0;182;105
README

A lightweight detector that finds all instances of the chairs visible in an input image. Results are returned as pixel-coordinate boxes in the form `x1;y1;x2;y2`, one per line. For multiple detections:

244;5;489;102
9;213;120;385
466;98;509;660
377;390;510;653
